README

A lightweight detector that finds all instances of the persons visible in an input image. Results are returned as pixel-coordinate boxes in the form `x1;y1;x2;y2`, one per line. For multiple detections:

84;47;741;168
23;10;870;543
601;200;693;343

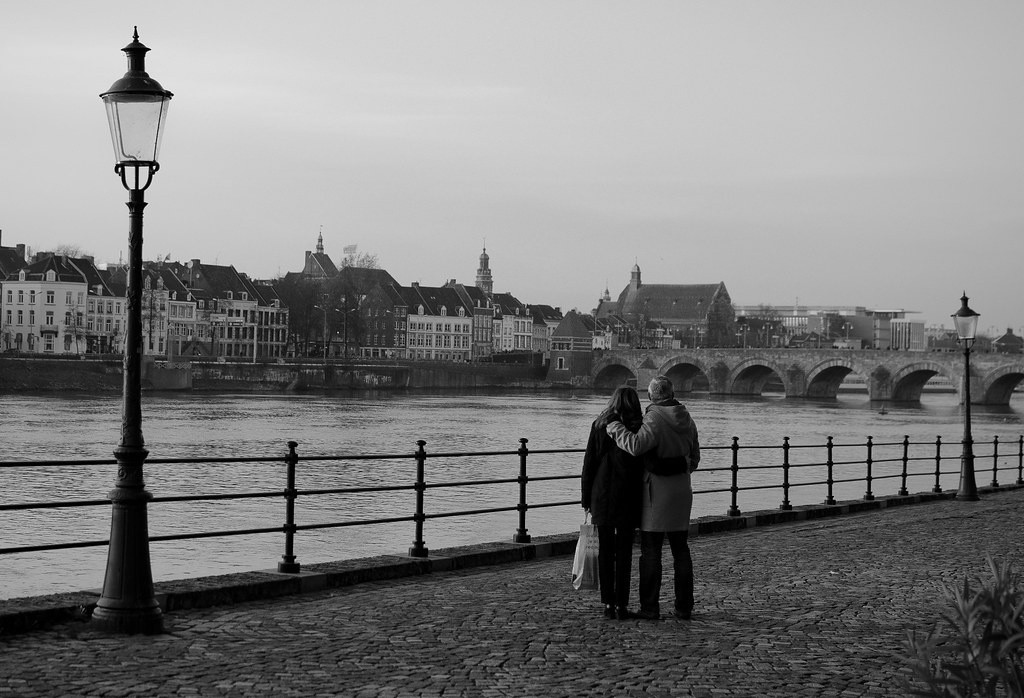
605;375;699;618
581;385;692;619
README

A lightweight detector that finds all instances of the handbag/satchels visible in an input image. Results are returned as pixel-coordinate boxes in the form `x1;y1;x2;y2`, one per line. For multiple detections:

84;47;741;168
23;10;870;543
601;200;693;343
571;511;599;592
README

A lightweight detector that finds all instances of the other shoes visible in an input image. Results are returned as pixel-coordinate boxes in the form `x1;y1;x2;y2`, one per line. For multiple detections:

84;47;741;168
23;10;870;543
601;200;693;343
603;604;616;620
630;608;660;619
615;606;632;621
672;606;693;618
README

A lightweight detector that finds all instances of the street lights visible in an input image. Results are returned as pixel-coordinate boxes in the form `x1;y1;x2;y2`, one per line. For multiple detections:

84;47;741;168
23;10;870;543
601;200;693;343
950;290;980;502
335;309;355;358
619;322;942;350
314;305;326;360
90;23;177;634
